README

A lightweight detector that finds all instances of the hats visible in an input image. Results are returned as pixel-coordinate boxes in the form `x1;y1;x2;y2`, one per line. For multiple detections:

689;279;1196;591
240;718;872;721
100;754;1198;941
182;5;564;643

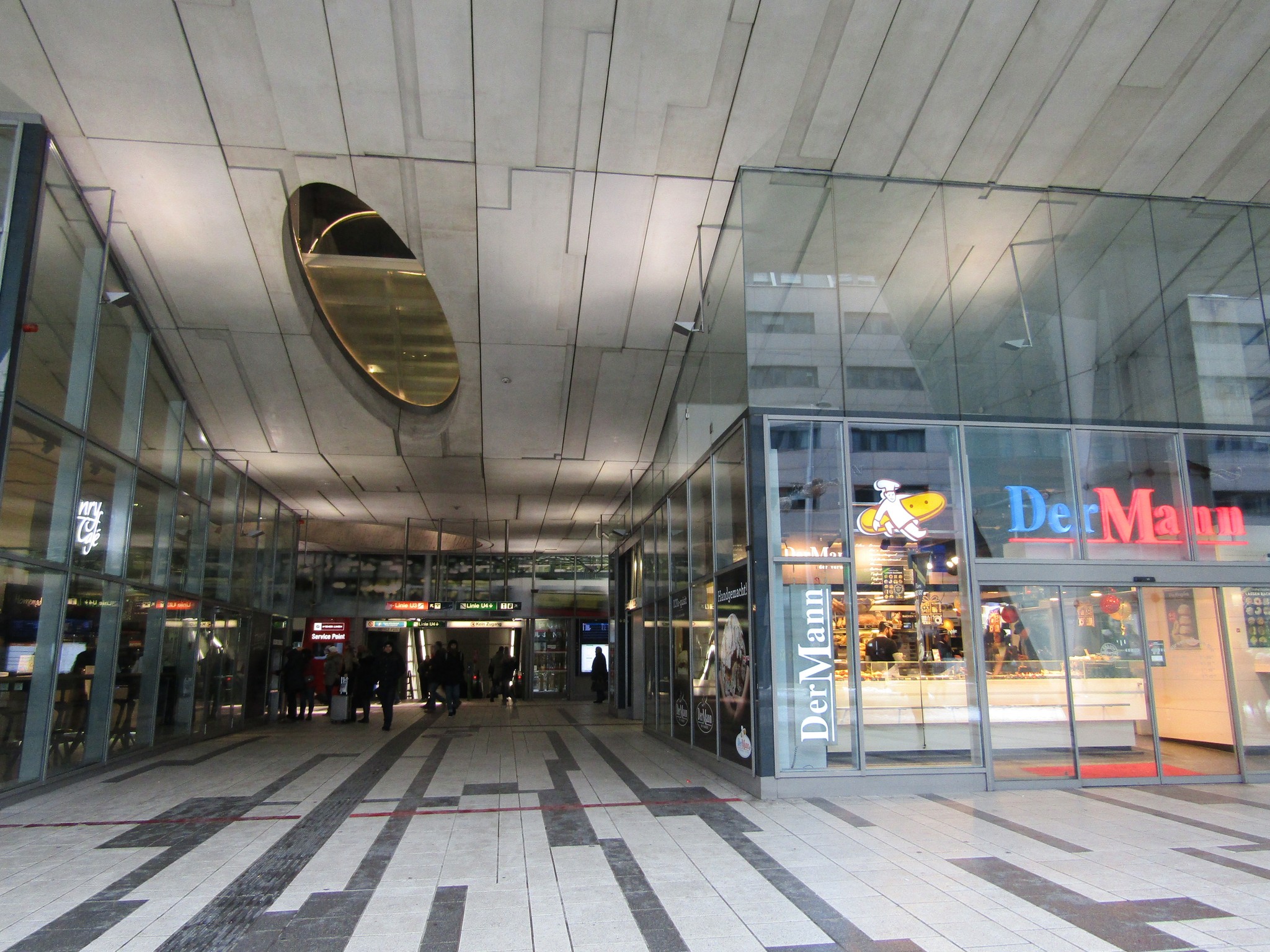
329;646;338;652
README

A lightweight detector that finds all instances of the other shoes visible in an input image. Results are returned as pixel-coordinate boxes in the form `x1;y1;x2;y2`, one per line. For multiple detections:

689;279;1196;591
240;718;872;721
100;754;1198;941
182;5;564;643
322;711;330;716
593;700;602;703
394;699;401;705
448;712;453;717
374;701;381;705
502;699;508;702
305;716;312;721
421;704;429;709
424;709;437;714
295;715;305;721
348;718;356;722
358;718;370;723
490;697;494;702
286;714;296;719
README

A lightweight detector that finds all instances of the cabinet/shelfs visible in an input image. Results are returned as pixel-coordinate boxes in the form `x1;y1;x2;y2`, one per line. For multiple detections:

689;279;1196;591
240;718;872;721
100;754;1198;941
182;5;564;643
833;591;960;664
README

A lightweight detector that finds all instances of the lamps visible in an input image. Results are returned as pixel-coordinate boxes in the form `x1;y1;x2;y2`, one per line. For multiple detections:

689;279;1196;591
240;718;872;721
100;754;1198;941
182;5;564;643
93;185;137;313
239;459;265;539
612;469;634;537
670;224;708;337
998;237;1043;353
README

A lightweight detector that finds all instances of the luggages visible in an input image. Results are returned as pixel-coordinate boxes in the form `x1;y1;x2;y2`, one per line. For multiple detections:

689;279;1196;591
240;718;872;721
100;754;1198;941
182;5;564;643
329;677;351;723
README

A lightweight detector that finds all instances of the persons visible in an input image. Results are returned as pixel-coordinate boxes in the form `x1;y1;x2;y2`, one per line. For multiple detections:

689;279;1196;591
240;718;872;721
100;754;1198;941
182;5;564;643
377;642;406;732
719;613;751;723
591;647;608;703
1013;621;1049;671
931;628;962;672
472;682;483;699
418;639;465;718
487;646;513;702
990;630;1015;674
273;642;376;724
394;691;401;704
865;621;905;678
